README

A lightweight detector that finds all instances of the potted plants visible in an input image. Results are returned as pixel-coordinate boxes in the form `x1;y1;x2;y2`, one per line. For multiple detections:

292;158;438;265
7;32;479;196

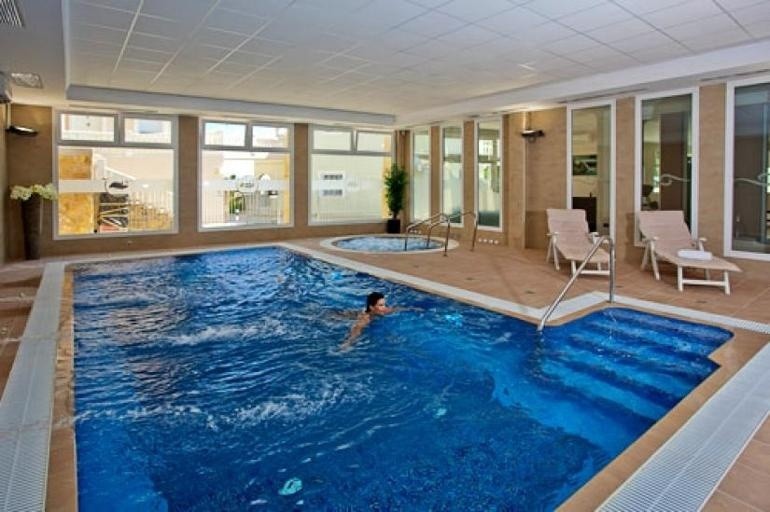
384;163;409;234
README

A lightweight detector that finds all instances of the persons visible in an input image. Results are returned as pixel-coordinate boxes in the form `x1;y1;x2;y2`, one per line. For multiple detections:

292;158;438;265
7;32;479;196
327;292;425;351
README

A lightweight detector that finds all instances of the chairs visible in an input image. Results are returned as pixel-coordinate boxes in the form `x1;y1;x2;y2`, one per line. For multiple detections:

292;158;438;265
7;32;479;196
545;208;743;297
